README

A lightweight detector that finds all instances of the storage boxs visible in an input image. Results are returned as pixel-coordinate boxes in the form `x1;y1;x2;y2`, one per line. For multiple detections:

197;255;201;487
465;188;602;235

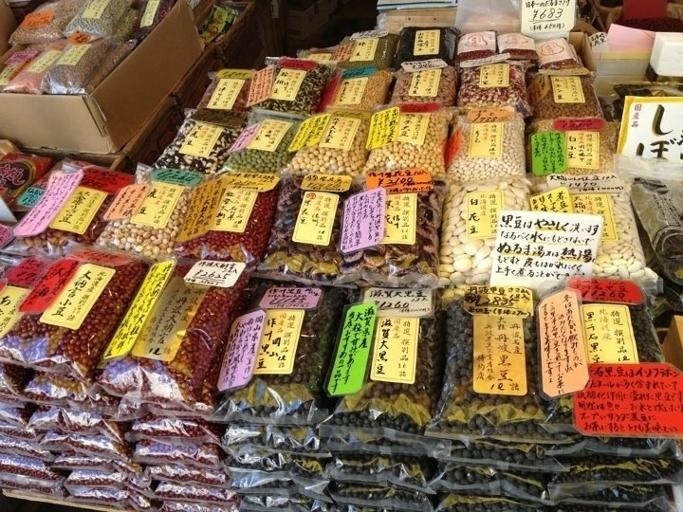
0;0;205;154
201;0;275;71
